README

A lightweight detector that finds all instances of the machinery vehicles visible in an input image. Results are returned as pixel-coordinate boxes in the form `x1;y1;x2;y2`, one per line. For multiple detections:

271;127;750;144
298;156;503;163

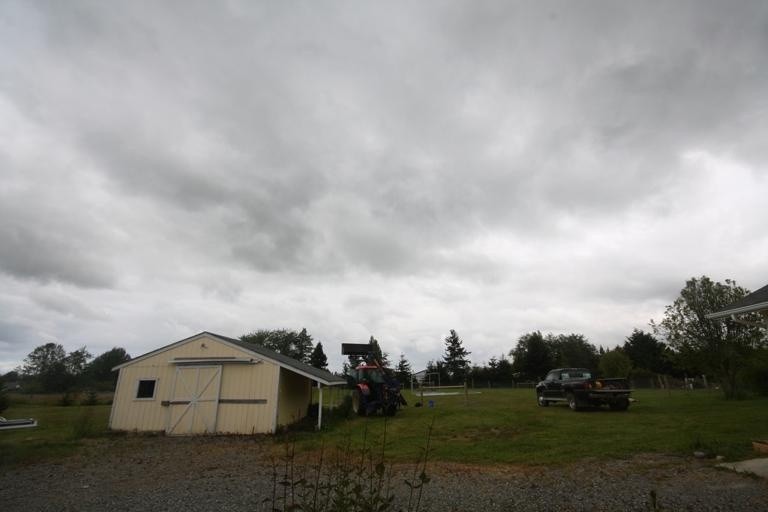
340;343;407;416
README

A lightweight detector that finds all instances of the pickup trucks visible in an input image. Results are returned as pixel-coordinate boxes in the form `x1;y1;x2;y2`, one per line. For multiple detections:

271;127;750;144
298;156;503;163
534;367;635;413
